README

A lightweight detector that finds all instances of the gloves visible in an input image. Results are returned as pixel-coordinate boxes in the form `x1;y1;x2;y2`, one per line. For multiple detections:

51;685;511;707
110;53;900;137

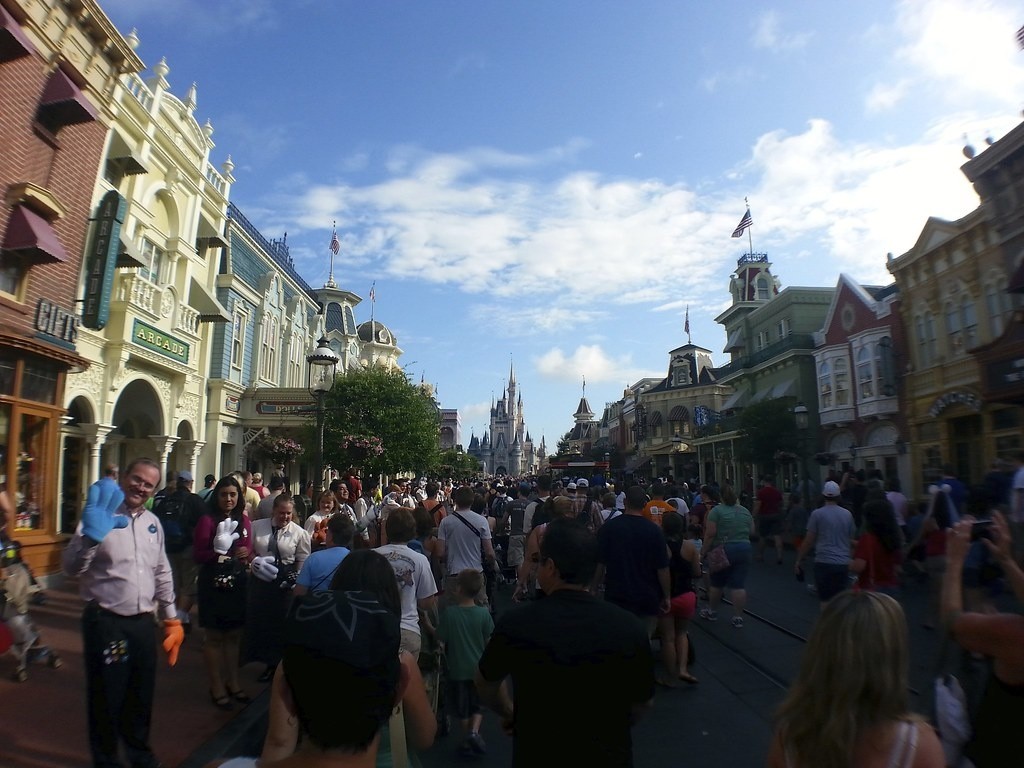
382;491;396;506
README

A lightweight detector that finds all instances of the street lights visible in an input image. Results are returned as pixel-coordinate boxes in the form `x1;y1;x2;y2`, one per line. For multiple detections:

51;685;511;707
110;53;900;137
792;400;810;515
306;334;339;512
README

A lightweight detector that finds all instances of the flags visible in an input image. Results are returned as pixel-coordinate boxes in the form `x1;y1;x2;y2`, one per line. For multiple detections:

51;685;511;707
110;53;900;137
731;209;753;237
369;286;376;302
684;311;689;333
329;229;340;255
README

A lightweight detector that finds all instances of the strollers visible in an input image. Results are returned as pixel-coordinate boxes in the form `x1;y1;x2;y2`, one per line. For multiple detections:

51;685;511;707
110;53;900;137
490;528;518;592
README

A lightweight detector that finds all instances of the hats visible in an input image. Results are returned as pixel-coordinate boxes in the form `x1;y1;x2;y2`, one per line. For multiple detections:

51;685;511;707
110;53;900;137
822;480;841;497
576;478;589;487
365;479;379;491
275;463;285;468
567;482;577;490
176;471;194;481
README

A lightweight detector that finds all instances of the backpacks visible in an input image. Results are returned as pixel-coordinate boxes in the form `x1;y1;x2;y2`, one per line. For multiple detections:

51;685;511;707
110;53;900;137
492;495;511;519
154;496;189;554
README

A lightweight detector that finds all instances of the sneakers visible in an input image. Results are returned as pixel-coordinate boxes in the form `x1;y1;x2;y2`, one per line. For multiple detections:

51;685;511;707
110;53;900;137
729;615;743;628
699;608;718;621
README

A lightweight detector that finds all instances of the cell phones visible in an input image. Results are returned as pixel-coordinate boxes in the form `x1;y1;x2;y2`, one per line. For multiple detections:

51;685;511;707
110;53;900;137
970;521;994;542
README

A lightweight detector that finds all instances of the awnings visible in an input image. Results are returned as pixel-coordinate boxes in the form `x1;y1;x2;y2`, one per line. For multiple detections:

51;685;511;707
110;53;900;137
108;128;148;177
3;203;64;266
0;6;31;63
720;381;793;412
190;276;232;322
197;213;230;248
626;457;652;474
41;67;95;125
648;406;688;426
115;233;146;268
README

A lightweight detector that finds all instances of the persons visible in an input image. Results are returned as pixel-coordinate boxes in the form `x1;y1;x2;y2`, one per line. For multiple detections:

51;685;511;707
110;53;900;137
0;489;61;682
753;467;1024;768
202;587;408;768
767;592;946;768
493;474;756;689
475;517;656;768
63;457;182;768
104;462;496;759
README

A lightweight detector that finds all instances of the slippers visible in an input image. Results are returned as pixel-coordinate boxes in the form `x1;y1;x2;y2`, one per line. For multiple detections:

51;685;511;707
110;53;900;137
677;670;699;684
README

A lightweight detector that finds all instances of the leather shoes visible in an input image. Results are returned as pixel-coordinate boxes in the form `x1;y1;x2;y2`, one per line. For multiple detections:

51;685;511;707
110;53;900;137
228;687;251;705
257;665;275;682
209;687;234;711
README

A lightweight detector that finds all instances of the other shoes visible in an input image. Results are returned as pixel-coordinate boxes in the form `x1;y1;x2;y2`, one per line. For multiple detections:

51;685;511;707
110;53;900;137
13;666;29;683
180;616;192;635
468;733;487;755
775;558;783;566
455;738;472;757
46;656;65;671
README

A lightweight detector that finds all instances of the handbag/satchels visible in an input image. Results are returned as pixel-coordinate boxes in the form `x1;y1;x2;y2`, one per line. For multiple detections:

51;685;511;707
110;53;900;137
0;621;13;654
703;543;731;577
